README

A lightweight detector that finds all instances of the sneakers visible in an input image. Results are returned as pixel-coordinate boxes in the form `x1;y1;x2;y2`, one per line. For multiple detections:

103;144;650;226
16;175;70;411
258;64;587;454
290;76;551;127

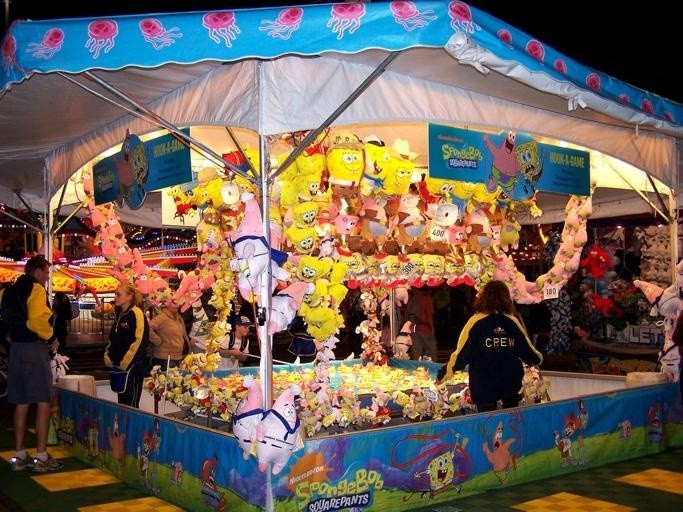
32;453;64;472
9;451;34;470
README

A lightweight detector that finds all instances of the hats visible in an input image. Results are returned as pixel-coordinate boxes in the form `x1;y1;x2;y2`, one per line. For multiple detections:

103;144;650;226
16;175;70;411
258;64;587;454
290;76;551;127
232;315;254;327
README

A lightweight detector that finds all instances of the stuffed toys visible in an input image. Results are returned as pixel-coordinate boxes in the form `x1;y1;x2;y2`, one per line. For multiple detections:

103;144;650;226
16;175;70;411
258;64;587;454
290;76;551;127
80;125;683;476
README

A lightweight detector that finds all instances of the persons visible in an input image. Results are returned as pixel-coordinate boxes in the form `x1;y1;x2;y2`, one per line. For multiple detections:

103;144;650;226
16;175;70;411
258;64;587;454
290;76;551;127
51;293;74;358
0;256;65;474
